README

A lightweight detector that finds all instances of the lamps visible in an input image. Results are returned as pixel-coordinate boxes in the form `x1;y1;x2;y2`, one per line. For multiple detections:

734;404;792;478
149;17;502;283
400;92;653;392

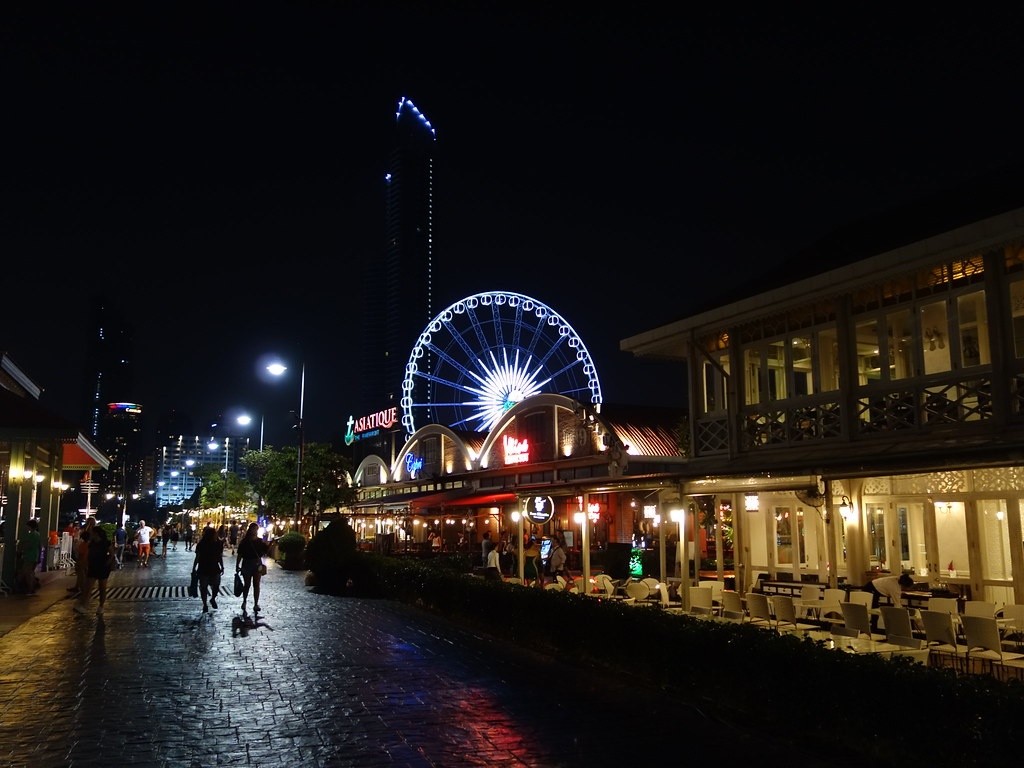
839;496;852;520
744;492;759;512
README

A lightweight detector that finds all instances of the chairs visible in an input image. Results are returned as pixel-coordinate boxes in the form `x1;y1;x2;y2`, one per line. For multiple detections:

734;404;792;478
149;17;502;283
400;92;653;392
501;573;1023;683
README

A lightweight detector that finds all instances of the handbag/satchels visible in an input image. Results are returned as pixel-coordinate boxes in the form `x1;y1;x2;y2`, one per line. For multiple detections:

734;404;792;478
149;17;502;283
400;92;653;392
234;573;243;597
104;554;120;571
191;572;197;596
259;564;266;576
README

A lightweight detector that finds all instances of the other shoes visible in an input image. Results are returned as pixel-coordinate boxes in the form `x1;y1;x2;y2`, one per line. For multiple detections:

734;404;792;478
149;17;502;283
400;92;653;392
203;605;208;613
66;587;80;594
73;605;88;613
96;606;104;615
242;602;246;609
254;604;261;612
210;598;217;609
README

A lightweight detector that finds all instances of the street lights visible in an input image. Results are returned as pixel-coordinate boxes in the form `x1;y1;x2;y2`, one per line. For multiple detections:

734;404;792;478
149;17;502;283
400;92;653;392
186;459;203;529
209;437;230;524
238;415;265;524
172;470;185;532
268;364;304;535
158;481;170;507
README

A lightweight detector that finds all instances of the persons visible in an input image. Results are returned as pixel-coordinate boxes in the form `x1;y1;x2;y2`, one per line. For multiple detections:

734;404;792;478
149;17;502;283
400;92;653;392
234;524;269;612
0;509;569;600
192;528;225;613
73;527;115;615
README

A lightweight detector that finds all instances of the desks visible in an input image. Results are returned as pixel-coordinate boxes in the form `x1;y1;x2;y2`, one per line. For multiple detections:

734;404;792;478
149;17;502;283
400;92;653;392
739;595;862;627
778;630;917;653
866;606;1015;648
